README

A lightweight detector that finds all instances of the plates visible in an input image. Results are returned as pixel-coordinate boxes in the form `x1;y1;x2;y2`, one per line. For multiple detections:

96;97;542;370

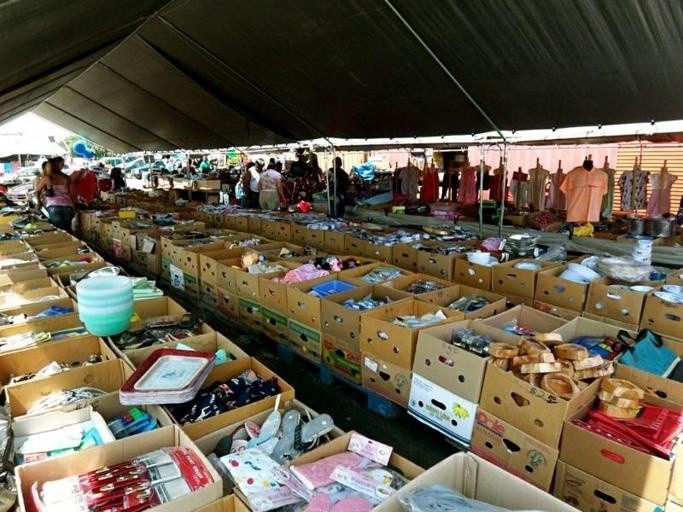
630;284;683;305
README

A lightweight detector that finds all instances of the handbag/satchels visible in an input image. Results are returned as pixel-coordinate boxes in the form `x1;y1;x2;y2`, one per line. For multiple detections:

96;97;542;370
43;186;54;197
610;327;683;383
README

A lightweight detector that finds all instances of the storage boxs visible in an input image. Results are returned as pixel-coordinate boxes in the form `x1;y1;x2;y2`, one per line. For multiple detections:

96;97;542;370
0;178;681;511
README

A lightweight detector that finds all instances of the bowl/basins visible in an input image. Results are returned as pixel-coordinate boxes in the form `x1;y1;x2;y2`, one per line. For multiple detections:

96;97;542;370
567;263;602;282
76;276;133;337
468;252;490;265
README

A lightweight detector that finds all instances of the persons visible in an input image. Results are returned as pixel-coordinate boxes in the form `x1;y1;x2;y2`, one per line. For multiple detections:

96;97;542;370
33;156;78;237
33;161;50;212
153;153;219;178
67;164;101;209
233;150;323;212
324;156;349;218
109;167;125;191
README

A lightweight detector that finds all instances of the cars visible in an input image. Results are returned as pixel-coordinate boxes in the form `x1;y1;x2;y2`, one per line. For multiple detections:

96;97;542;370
0;167;42;202
98;151;186;179
